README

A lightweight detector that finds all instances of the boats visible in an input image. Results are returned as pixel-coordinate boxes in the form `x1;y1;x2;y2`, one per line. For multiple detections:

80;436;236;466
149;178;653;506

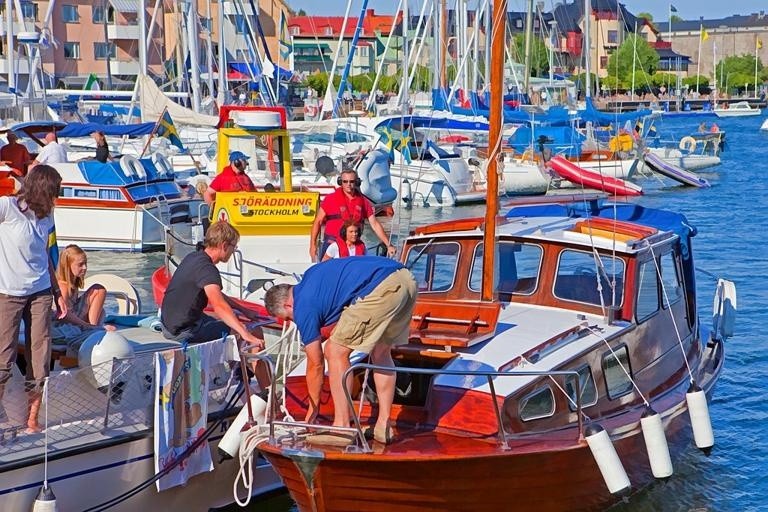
712;101;762;116
0;289;305;512
248;0;726;512
0;244;369;512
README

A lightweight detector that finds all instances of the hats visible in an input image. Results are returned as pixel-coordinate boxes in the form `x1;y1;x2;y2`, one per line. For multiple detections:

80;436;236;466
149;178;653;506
229;151;249;161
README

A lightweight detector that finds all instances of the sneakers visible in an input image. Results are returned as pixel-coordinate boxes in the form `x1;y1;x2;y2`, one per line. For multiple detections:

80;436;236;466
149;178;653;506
315;427;356;442
362;425;393;443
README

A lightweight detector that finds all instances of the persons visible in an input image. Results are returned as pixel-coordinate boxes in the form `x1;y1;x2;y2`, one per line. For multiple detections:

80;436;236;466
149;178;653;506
160;222;280;417
49;244;116;354
0;132;32;178
264;256;418;447
309;170;397;263
77;132;116;163
0;165;67;434
319;219;368;264
23;132;67;180
203;151;258;213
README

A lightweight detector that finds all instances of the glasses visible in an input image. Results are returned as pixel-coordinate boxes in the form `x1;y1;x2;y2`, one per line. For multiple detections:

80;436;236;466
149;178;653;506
227;242;238;248
342;179;357;183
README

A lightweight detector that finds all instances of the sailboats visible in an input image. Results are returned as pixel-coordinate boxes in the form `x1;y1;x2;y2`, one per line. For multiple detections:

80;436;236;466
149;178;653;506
236;195;739;512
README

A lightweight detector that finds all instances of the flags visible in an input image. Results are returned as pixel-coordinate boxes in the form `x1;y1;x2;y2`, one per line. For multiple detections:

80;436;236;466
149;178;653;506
635;123;643;136
280;12;288;34
379;125;395;166
82;74;101;100
670;4;677;12
153;109;188;153
700;29;708;45
279;40;293;60
395;130;412;166
756;40;760;50
647;126;656;138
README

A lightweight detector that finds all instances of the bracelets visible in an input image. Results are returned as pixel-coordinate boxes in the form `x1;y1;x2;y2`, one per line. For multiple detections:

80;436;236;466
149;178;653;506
52;288;59;296
